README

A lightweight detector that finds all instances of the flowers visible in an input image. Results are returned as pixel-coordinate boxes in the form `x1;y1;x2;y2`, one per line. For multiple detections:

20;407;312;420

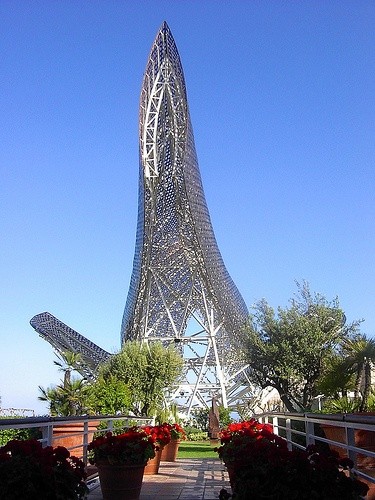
0;418;368;500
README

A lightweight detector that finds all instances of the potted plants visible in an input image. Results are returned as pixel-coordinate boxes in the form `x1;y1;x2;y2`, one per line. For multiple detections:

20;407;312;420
313;330;375;464
32;349;100;462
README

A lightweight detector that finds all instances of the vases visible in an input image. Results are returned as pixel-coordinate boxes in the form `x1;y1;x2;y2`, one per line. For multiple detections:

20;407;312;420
158;437;182;462
144;446;166;475
92;461;148;500
